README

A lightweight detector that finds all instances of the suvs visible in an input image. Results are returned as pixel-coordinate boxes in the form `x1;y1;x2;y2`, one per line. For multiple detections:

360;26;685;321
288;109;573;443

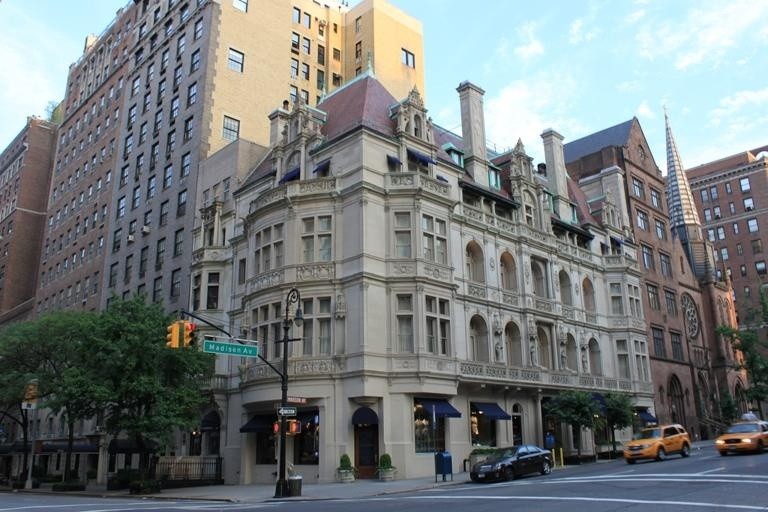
623;424;692;465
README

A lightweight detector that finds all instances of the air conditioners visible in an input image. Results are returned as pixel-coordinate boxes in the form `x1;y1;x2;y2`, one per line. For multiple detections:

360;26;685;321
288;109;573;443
127;235;134;241
141;226;150;233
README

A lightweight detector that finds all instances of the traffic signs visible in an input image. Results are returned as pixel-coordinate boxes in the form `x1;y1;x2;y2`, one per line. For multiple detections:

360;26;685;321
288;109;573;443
204;340;258;359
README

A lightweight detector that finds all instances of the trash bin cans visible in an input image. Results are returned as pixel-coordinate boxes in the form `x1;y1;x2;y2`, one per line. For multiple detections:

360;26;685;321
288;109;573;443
288;479;301;497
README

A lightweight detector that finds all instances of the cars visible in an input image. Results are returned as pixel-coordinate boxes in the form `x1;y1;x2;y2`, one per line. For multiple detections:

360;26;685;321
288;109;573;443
470;445;554;484
715;413;767;456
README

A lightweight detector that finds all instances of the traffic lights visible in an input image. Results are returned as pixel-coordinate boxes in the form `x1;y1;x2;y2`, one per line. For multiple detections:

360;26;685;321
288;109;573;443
274;423;279;433
166;320;195;349
289;420;300;433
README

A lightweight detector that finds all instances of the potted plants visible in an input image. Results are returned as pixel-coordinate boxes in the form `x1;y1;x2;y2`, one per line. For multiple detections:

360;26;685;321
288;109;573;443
471;449;488;466
337;454;356;482
375;454;396;480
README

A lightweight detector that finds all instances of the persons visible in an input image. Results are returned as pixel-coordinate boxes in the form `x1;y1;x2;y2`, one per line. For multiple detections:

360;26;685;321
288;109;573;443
544;430;556;461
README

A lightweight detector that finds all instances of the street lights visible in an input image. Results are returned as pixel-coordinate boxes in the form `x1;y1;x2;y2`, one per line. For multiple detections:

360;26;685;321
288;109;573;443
274;289;303;498
